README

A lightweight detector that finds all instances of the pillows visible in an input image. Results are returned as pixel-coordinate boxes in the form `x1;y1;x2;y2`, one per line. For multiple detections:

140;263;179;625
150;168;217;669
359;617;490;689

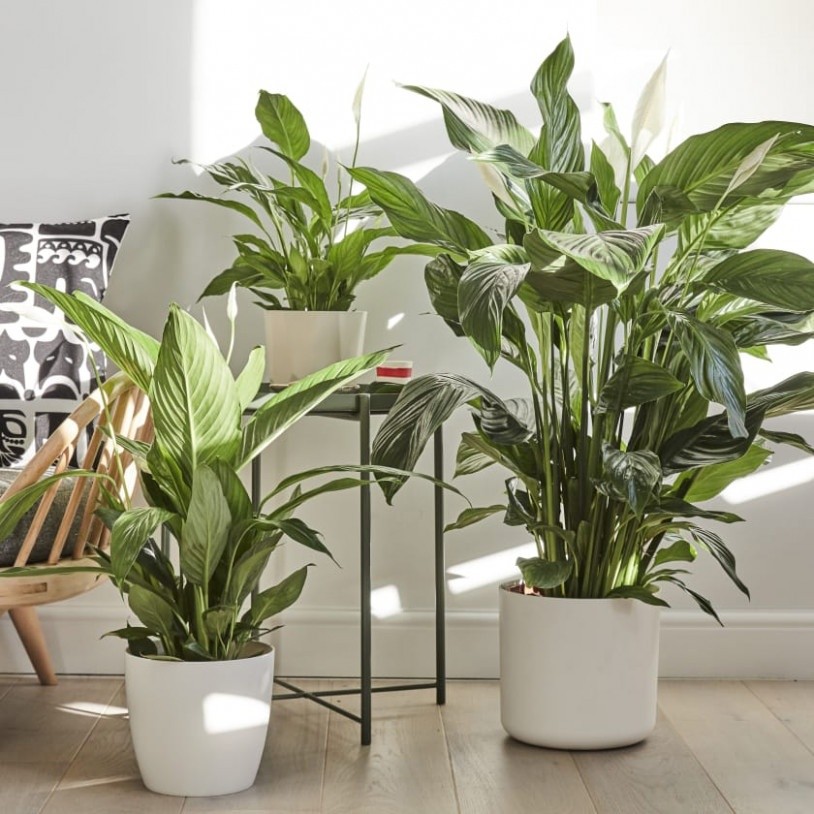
0;212;131;469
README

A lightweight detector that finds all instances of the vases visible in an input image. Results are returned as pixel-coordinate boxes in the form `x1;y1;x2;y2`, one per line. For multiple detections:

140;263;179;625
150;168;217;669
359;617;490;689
262;308;371;389
119;643;281;792
492;571;669;747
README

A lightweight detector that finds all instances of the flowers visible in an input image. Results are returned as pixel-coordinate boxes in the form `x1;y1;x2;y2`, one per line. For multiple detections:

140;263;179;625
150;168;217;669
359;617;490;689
347;31;814;594
2;264;375;660
151;63;439;309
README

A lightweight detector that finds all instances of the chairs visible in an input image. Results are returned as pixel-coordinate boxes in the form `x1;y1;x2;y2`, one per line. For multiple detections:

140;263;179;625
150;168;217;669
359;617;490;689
0;363;159;685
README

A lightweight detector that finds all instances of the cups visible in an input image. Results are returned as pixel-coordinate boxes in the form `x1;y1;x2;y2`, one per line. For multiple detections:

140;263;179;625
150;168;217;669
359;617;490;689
377;360;413;386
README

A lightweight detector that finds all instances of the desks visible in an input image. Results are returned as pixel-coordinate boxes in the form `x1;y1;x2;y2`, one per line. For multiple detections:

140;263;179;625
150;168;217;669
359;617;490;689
234;385;446;747
